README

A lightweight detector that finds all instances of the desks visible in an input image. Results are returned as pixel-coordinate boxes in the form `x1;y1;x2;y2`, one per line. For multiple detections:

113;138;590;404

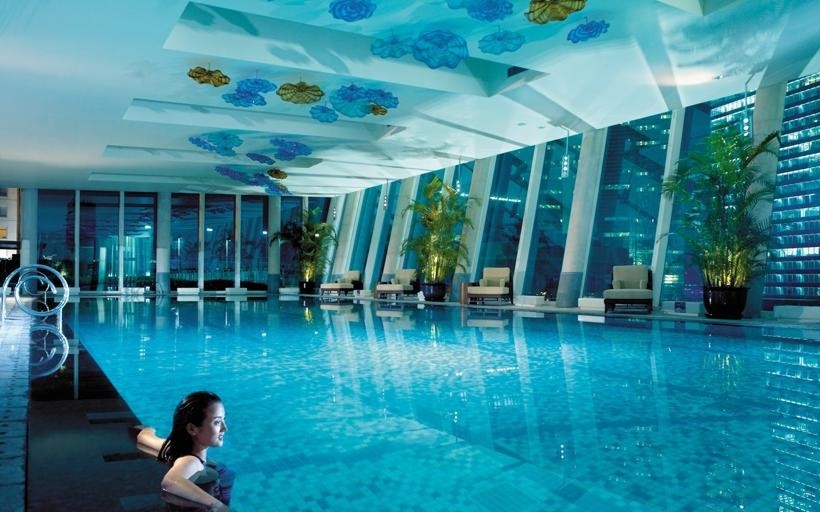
578;297;604;310
514;310;543;318
516;294;544;307
577;314;605;324
354;289;372;297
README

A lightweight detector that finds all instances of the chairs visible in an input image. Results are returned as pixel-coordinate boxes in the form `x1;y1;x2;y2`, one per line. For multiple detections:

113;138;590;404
604;265;654;315
373;303;417;331
466;307;510;344
315;299;361;323
374;268;421;300
319;271;361;298
467;267;514;305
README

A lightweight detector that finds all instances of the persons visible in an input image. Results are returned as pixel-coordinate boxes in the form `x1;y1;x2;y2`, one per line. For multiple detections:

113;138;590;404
135;390;231;512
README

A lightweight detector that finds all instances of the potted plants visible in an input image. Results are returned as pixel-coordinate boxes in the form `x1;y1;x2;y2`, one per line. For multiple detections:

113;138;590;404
421;305;450;343
398;175;481;302
696;322;750;398
659;123;785;319
264;198;339;294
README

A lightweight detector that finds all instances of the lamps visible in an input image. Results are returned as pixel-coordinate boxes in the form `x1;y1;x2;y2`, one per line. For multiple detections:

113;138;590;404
555;125;570;178
383;178;389;208
332;198;337;220
455;156;463;194
740;72;758;138
